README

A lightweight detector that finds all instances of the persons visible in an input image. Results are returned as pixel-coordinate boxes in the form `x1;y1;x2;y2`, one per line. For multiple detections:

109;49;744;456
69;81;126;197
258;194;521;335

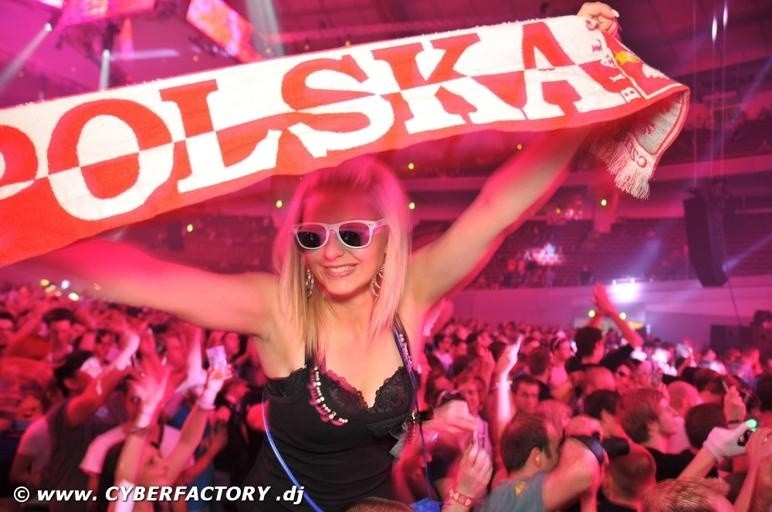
1;3;625;511
0;280;771;512
474;247;594;286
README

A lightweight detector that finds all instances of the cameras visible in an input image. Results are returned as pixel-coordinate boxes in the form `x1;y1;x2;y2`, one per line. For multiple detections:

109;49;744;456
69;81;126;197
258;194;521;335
593;431;629;458
737;429;753;446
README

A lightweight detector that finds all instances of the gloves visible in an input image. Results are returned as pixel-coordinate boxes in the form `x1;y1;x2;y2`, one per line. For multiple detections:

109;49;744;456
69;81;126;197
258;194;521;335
702;421;751;463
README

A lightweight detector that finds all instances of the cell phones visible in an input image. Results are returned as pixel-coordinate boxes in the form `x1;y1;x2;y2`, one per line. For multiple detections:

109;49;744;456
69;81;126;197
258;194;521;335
722;380;730;392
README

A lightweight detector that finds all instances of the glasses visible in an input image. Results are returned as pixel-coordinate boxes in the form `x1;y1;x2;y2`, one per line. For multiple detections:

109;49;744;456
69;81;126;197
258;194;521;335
291;217;391;249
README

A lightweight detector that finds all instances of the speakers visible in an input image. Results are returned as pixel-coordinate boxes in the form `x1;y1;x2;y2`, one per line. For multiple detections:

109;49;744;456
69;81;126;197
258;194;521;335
685;197;727;288
